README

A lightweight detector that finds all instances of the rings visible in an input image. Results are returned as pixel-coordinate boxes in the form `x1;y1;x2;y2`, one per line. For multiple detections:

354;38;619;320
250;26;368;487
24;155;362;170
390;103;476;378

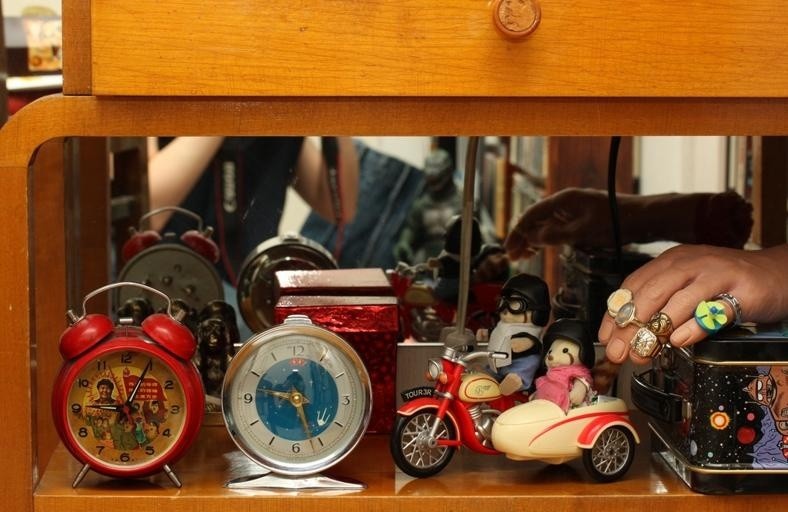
605;285;632;316
614;302;646;328
645;308;678;344
692;298;728;332
712;291;742;328
632;327;664;357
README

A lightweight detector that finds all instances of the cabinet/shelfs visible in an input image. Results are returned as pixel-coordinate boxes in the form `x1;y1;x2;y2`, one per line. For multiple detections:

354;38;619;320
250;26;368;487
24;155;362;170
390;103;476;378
0;0;788;512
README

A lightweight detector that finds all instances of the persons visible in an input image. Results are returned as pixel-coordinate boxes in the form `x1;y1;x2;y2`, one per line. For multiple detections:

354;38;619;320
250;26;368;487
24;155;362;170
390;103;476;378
145;137;358;344
505;187;751;265
595;239;787;364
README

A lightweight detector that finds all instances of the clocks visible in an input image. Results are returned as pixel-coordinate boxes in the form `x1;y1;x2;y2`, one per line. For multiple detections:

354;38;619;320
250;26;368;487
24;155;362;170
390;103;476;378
52;282;205;489
220;315;372;492
110;206;224;315
235;232;342;330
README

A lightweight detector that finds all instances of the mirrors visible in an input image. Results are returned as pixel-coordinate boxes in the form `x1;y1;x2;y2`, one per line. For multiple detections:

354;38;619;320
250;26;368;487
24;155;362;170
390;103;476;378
67;137;784;425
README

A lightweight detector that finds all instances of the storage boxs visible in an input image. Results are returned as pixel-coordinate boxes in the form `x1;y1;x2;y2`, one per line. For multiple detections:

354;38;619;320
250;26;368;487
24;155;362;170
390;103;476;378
548;244;659;341
631;323;788;494
